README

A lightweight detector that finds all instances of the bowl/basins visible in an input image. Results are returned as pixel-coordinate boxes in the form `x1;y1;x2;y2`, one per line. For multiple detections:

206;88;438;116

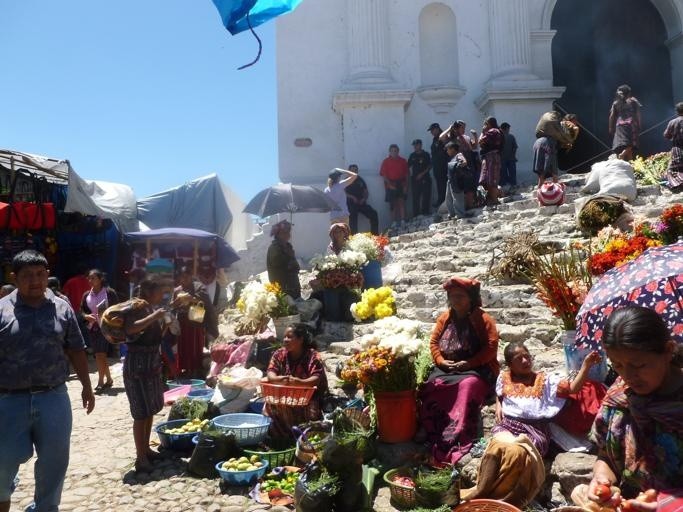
163;378;216;407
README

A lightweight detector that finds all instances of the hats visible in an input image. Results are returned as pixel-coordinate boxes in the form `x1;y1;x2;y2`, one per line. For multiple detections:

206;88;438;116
328;169;342;178
411;139;421;145
426;123;438;130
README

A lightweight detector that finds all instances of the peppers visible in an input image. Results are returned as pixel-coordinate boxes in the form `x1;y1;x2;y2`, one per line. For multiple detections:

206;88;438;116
258;468;299;495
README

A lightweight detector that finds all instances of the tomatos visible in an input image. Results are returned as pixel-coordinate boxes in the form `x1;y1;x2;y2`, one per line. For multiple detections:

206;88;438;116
595;483;658;512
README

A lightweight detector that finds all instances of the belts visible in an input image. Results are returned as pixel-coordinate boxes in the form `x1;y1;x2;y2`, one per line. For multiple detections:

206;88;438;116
0;385;56;393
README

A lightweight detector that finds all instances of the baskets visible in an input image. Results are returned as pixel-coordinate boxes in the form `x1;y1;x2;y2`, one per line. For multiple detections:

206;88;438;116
163;379;215;404
239;441;295;472
290;418;363;465
451;499;522;512
256;380;316;409
150;418;214;452
382;465;451;508
212;413;270;446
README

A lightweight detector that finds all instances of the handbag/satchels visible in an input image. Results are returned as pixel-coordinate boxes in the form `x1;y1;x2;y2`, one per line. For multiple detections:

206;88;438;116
187;301;205;323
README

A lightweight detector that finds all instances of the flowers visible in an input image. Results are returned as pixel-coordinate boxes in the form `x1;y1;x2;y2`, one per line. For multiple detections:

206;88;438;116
237;278;297;320
516;204;683;329
309;231;396;323
334;318;425;389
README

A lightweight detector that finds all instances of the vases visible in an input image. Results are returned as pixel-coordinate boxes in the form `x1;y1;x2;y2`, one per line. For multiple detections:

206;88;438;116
273;315;302;339
373;389;417;442
561;331;607;382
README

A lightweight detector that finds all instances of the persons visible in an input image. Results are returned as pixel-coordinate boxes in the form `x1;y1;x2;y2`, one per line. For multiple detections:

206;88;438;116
46;262;228;474
379;116;519;227
571;305;683;512
462;343;602;510
266;220;301;299
608;85;642;161
325;165;379;236
0;249;96;512
261;323;330;427
533;113;580;187
414;276;500;467
326;222;352;256
663;103;683;174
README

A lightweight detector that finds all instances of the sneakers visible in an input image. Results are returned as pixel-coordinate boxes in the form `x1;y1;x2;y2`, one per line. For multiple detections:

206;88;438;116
390;221;396;228
400;220;405;225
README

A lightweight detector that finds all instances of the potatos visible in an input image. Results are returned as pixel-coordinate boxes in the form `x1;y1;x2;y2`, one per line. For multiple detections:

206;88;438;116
222;454;263;473
164;417;209;435
